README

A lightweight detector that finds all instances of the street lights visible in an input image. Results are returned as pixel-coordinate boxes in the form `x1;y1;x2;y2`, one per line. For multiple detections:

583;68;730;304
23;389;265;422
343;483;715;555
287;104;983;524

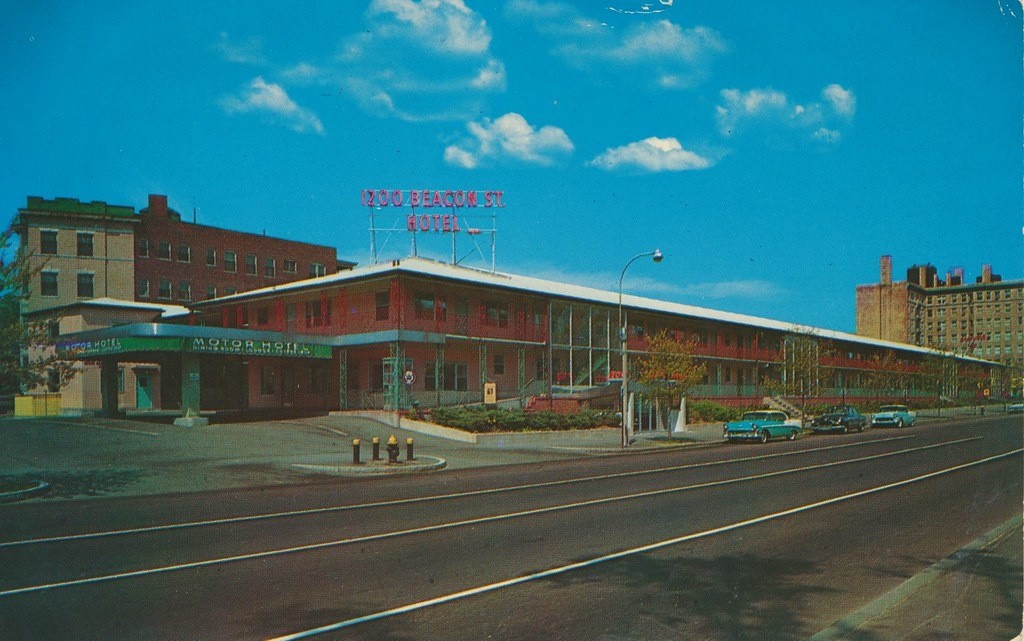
620;248;663;447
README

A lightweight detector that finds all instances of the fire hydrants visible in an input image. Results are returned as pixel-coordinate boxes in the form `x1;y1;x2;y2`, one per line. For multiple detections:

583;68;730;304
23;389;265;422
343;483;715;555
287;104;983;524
386;435;400;463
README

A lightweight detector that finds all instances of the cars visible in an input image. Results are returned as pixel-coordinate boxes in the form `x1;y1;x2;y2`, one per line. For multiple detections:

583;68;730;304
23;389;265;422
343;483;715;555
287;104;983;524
722;409;803;444
810;404;867;435
871;405;917;429
1007;401;1024;413
